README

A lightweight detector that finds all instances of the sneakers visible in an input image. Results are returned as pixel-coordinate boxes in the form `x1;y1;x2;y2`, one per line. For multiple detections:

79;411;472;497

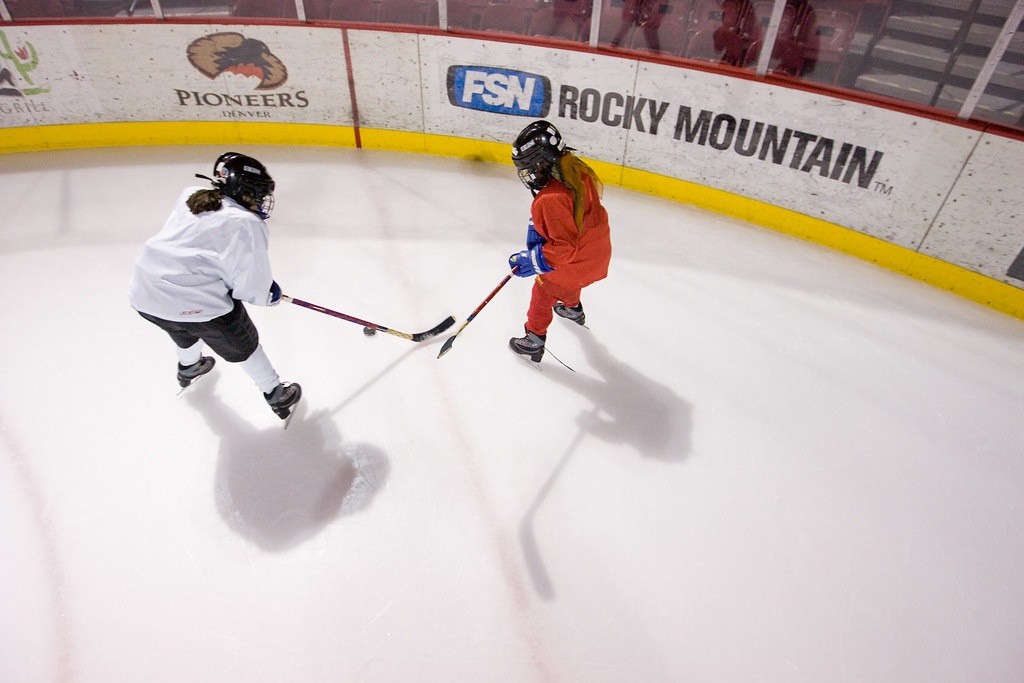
509;323;547;372
264;383;302;430
554;302;590;330
176;352;216;397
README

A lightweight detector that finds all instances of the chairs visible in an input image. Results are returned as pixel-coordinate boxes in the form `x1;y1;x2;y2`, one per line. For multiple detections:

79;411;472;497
230;0;897;87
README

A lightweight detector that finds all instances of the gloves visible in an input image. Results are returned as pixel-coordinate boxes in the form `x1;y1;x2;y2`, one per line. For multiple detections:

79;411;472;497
509;244;556;278
527;218;547;249
270;281;282;306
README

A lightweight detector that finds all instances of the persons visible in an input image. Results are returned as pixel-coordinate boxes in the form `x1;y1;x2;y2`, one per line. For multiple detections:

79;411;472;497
129;151;301;419
547;0;819;76
508;120;612;362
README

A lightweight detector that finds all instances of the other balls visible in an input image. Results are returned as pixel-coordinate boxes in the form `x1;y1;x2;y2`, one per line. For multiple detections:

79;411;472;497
363;326;377;337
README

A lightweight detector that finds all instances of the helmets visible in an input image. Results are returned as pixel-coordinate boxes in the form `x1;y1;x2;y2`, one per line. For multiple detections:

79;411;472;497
214;152;275;208
512;120;566;170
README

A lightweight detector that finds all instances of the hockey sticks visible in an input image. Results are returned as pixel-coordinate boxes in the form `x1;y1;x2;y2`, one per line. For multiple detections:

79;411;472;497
436;265;520;358
280;294;456;342
